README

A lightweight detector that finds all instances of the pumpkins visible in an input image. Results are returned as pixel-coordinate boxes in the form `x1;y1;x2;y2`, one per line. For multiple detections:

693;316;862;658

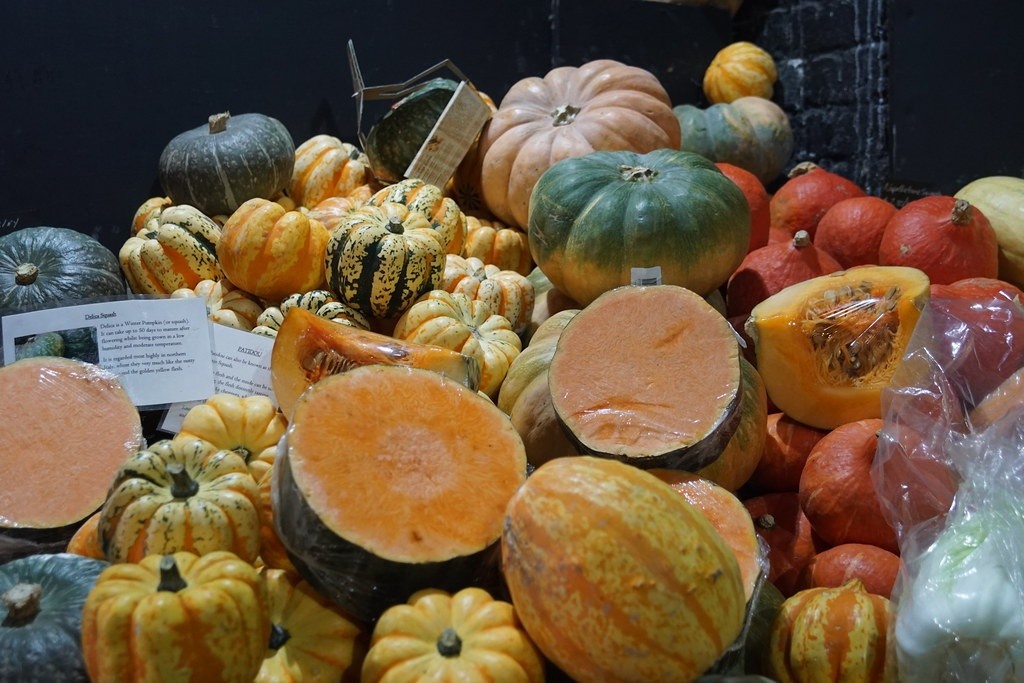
0;41;1024;683
